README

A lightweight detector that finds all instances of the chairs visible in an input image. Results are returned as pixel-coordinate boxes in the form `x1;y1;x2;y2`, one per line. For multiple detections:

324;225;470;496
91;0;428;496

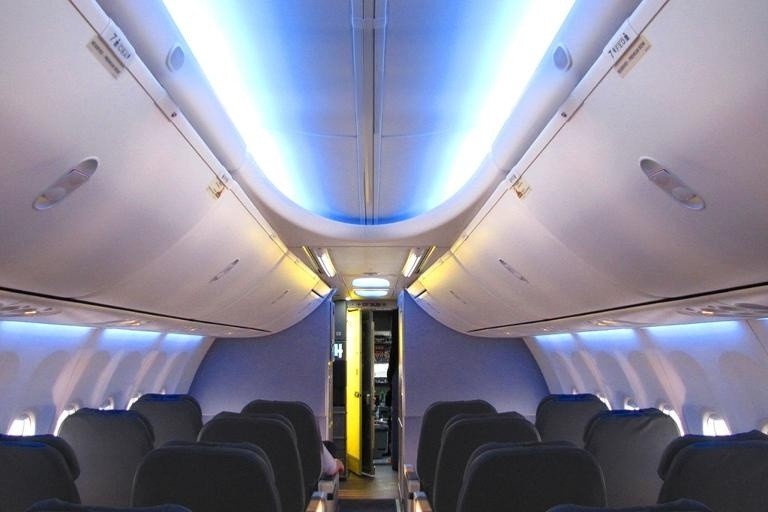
655;429;767;510
402;398;496;509
127;393;205;445
196;411;329;512
455;439;609;510
57;408;155;507
585;408;680;510
2;432;83;511
536;394;609;444
412;410;542;512
129;440;282;511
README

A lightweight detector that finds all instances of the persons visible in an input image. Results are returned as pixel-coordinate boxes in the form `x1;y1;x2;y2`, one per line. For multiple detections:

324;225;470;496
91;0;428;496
381;380;392;457
320;443;345;501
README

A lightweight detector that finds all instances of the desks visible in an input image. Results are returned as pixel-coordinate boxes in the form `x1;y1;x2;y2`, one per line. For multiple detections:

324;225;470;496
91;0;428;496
241;398;338;512
